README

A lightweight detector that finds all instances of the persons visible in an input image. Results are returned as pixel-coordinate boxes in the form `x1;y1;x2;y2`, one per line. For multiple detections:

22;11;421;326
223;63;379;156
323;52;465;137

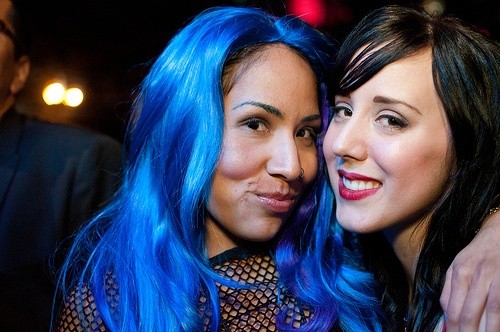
323;1;500;332
47;7;499;332
1;1;129;332
